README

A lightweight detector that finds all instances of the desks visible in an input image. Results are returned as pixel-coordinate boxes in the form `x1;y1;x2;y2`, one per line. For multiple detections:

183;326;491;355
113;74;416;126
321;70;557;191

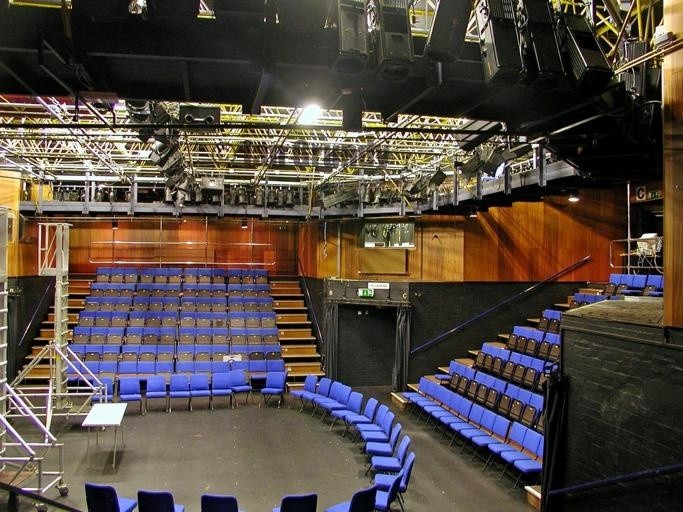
80;401;127;473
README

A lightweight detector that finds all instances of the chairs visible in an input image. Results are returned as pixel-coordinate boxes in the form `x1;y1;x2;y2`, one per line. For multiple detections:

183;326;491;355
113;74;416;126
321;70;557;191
288;375;416;512
80;481;318;512
401;272;665;491
61;265;289;414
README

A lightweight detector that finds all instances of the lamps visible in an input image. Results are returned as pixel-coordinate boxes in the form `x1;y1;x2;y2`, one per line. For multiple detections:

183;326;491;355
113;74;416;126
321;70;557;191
111;216;118;230
240;218;247;229
326;2;619;99
402;141;532;196
134;103;190;193
